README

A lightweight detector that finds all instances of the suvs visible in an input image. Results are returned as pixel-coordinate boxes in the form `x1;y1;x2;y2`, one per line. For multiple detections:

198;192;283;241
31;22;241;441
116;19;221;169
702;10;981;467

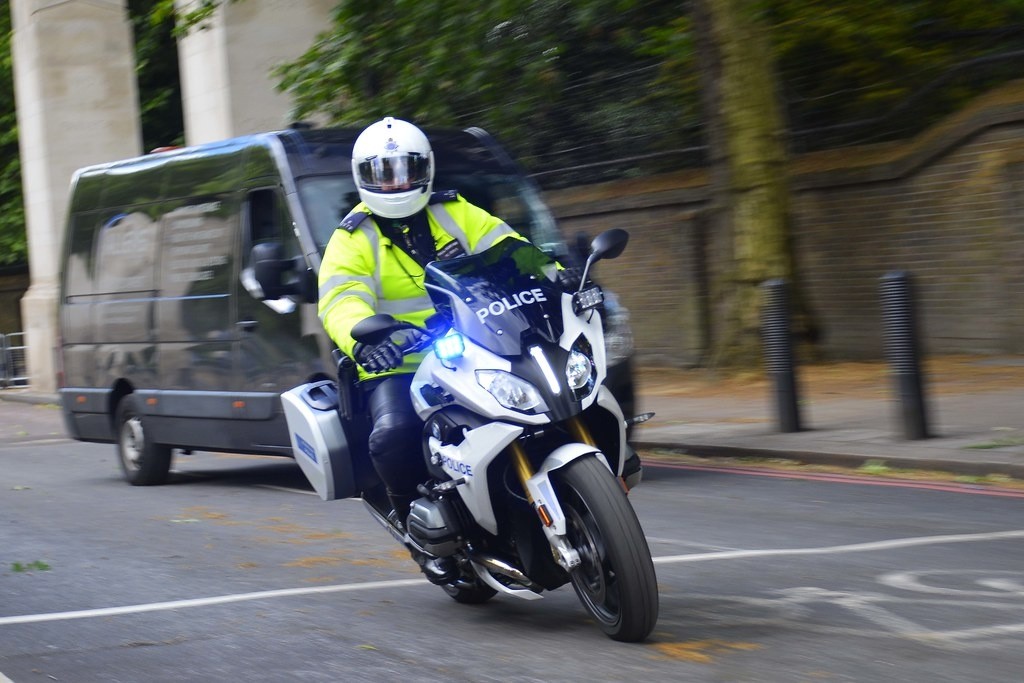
53;125;640;488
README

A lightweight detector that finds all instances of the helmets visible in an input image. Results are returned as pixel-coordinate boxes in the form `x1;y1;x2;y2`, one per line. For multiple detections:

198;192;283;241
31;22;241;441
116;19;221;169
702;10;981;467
349;116;434;218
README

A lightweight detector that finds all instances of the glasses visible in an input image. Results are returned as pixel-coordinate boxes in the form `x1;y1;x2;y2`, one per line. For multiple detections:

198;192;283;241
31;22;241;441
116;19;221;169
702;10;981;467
374;166;416;181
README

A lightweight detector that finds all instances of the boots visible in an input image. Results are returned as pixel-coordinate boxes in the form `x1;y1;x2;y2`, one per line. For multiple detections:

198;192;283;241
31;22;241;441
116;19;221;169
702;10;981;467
387;490;460;585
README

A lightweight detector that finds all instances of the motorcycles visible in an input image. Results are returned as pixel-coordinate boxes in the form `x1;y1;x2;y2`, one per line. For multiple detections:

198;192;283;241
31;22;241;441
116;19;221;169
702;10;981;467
280;225;660;644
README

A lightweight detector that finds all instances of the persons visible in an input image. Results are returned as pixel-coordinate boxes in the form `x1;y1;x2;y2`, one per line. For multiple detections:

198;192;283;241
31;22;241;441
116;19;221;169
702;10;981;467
318;117;641;585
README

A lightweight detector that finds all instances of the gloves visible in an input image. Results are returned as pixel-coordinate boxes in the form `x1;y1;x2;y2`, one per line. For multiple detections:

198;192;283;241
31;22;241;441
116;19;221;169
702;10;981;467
351;335;404;373
557;263;594;294
